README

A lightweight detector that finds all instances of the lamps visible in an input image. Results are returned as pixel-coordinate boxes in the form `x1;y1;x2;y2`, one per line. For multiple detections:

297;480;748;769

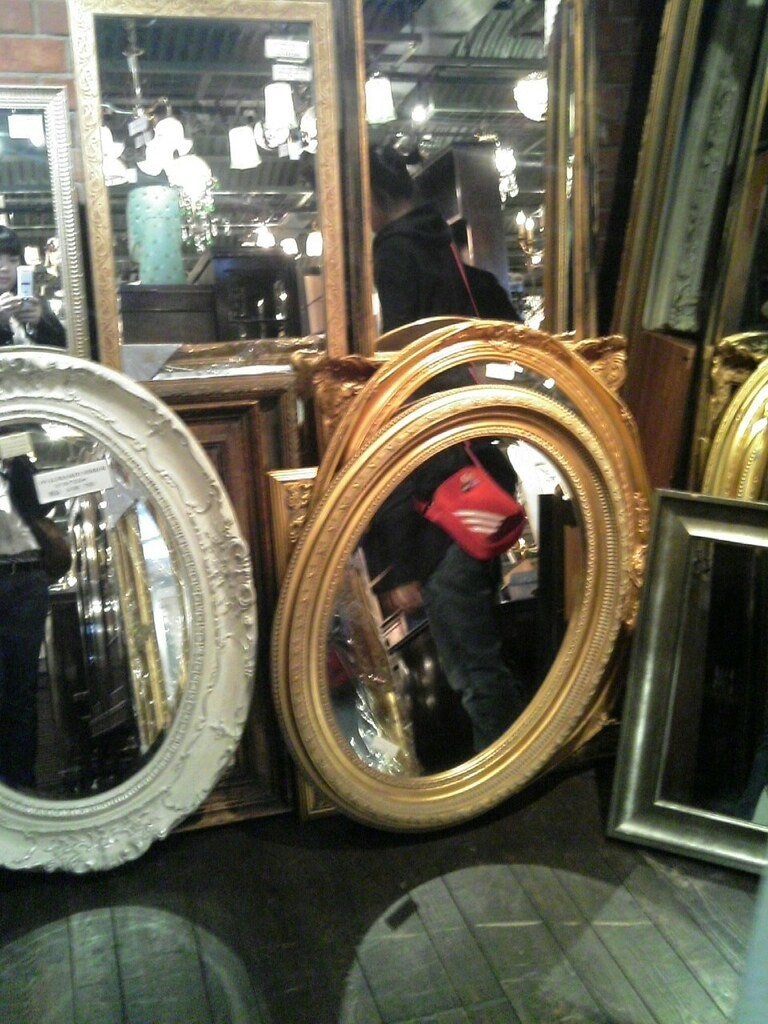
100;34;324;255
364;67;400;129
412;99;435;123
494;140;521;203
512;71;548;123
516;210;542;266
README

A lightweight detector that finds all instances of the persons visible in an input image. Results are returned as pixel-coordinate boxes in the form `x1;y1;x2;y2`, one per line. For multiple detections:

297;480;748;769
351;139;529;331
367;434;528;767
0;455;75;795
1;227;66;350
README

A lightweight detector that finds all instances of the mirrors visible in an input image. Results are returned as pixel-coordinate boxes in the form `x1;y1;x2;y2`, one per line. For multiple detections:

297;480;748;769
347;0;572;361
67;1;347;406
2;85;93;360
0;352;258;878
603;488;768;875
613;0;768;354
270;380;632;836
306;316;655;790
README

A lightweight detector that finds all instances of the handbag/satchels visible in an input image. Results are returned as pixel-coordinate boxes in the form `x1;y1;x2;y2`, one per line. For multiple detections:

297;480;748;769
431;465;528;562
